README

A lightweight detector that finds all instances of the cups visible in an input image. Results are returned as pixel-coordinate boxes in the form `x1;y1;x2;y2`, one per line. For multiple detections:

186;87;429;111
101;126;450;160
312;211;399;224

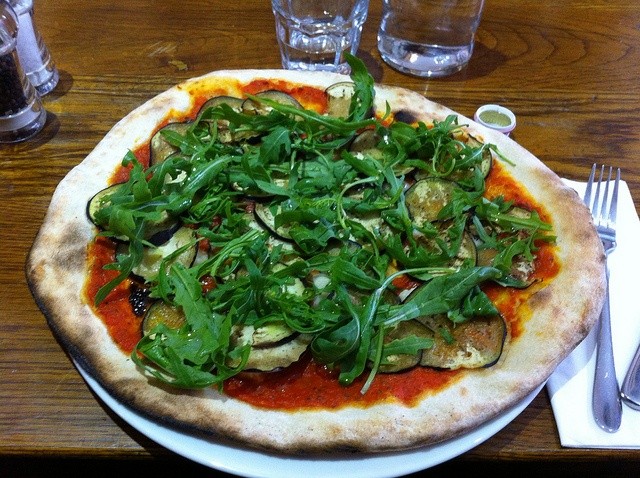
271;1;369;75
377;0;482;77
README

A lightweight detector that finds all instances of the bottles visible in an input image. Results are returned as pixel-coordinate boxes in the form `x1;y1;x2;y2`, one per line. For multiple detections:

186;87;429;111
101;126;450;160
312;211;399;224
0;1;48;144
1;1;60;97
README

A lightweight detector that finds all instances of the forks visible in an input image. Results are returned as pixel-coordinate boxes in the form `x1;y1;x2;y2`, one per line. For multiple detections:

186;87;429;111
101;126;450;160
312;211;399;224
584;163;623;433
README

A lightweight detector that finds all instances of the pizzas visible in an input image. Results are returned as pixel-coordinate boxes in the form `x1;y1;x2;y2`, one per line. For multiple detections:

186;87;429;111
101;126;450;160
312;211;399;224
25;69;608;456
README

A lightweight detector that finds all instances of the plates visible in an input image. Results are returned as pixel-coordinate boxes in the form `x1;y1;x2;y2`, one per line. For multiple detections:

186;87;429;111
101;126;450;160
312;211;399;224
65;349;549;477
